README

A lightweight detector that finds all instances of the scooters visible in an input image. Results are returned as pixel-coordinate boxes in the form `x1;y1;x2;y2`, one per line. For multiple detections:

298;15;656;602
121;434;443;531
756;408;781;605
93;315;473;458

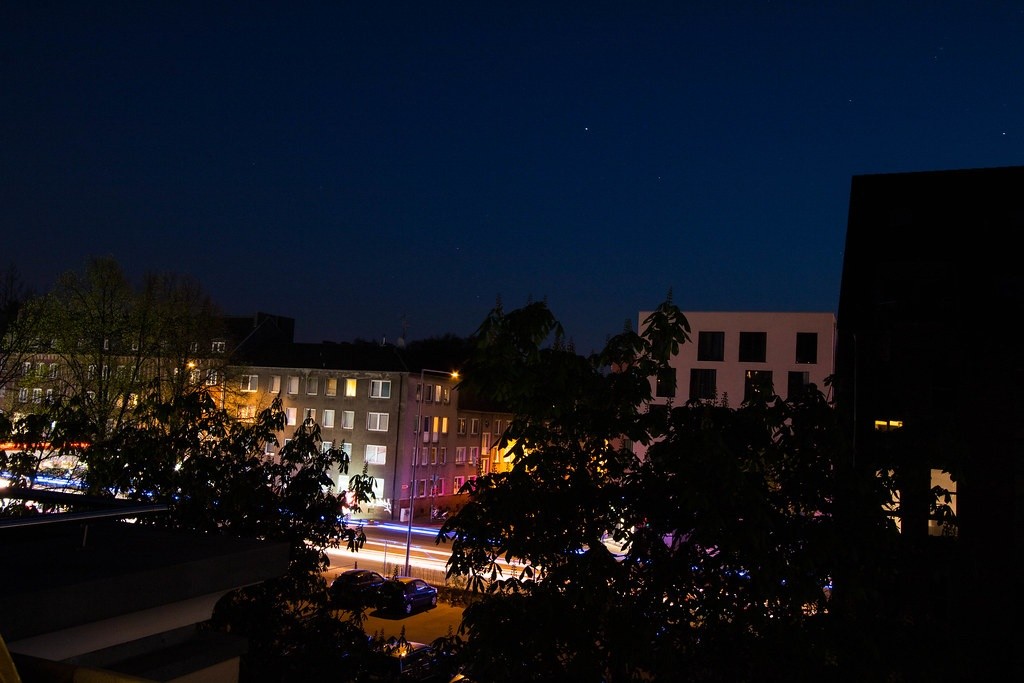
430;507;449;523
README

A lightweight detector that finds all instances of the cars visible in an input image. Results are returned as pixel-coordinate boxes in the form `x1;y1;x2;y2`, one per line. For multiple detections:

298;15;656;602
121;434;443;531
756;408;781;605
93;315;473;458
376;577;439;614
333;570;389;600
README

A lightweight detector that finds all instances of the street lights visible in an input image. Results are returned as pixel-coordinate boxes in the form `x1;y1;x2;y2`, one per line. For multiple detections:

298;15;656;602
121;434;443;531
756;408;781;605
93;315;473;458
403;370;459;577
